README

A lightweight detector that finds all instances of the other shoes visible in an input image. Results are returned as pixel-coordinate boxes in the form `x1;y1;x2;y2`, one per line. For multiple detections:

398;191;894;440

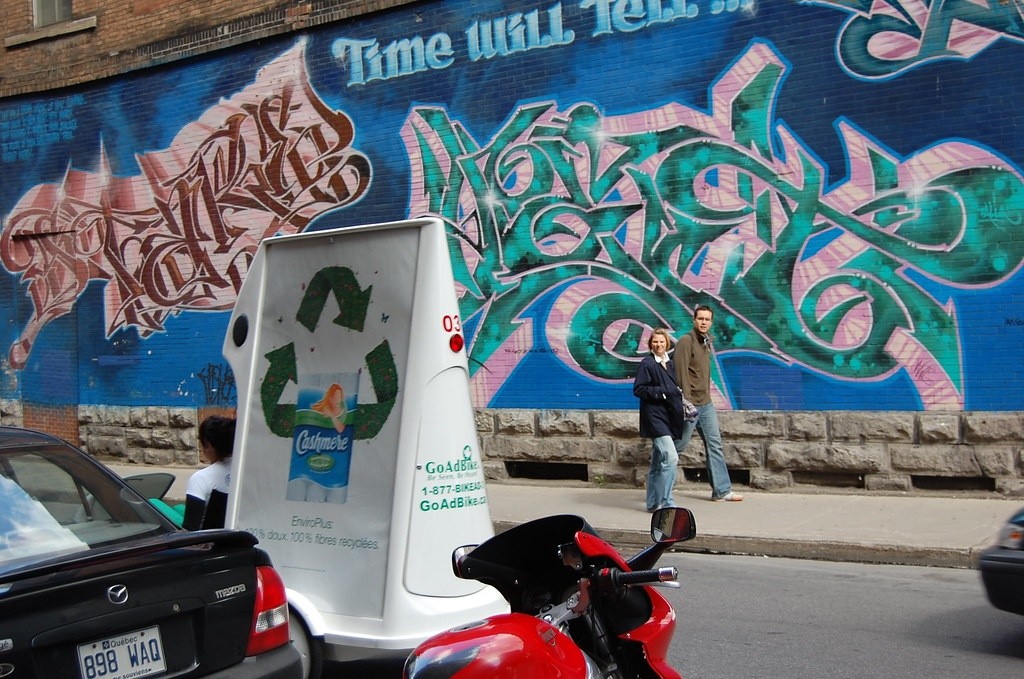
647;505;661;513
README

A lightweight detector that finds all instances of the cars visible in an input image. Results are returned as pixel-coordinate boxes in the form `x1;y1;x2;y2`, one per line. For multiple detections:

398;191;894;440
0;426;305;679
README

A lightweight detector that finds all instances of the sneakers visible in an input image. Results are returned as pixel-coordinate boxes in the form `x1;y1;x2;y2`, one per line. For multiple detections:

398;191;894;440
711;492;743;502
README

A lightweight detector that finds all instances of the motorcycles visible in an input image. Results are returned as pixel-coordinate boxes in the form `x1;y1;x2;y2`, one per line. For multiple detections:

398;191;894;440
403;505;697;678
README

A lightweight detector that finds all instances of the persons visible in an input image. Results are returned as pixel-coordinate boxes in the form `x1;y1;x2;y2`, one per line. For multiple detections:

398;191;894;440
182;415;236;531
633;328;685;513
672;306;743;502
